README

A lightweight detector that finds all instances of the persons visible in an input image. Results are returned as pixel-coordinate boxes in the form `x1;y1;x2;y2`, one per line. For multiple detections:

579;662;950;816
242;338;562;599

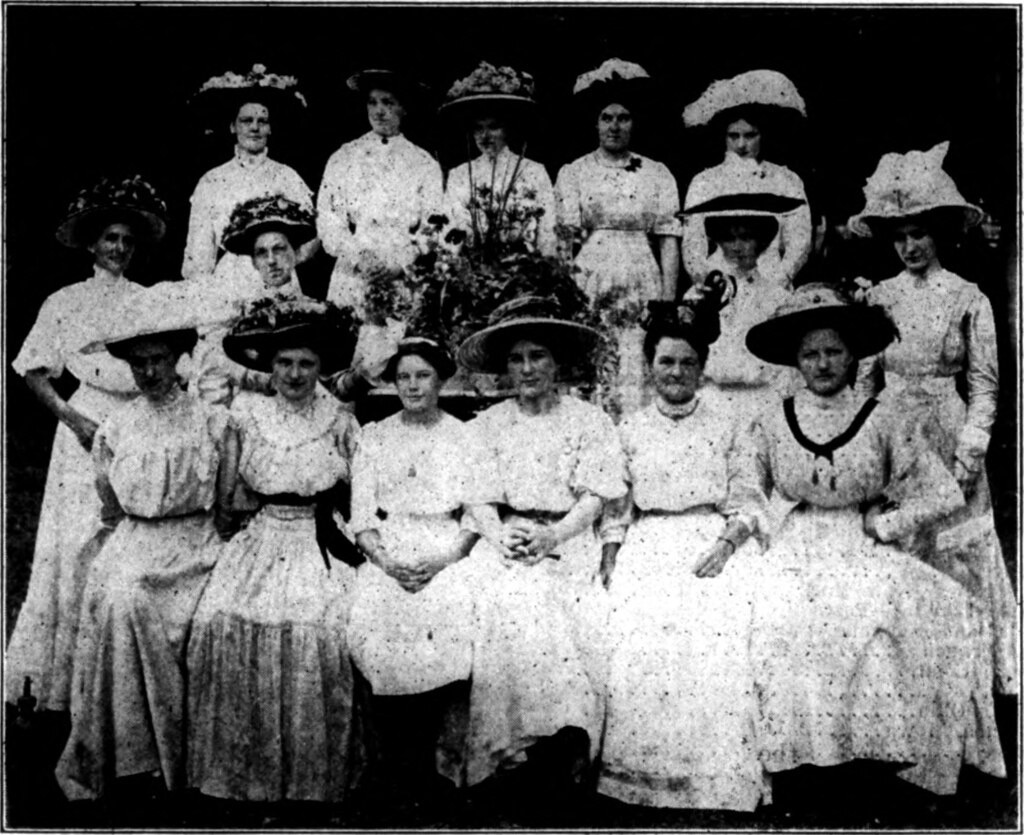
181;64;320;294
550;58;681;300
676;194;807;423
316;65;444;373
55;288;236;801
201;196;397;401
851;149;1019;698
435;64;555;255
3;178;166;712
189;283;1010;808
679;70;812;287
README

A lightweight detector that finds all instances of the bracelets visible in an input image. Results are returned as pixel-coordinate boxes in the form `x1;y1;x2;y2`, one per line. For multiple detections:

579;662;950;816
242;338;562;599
718;537;735;553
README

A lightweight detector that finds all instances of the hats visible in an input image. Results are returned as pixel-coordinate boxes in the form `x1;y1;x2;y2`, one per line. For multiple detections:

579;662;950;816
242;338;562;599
81;289;235;355
347;68;432;113
682;69;809;140
437;64;539;124
223;295;357;374
571;58;662;134
677;193;807;216
220;198;316;253
186;70;308;135
55;175;169;249
848;141;984;238
459;296;610;374
746;282;901;366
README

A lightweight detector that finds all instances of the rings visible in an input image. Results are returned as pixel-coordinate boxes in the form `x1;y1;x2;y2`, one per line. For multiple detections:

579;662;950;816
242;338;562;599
714;558;718;562
